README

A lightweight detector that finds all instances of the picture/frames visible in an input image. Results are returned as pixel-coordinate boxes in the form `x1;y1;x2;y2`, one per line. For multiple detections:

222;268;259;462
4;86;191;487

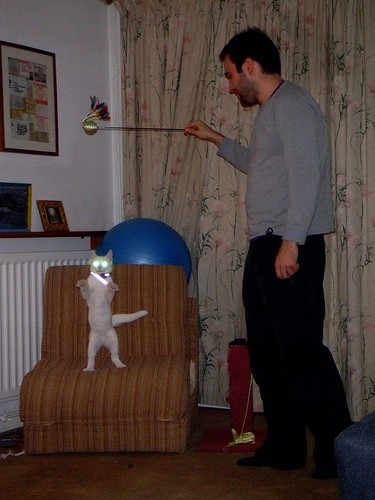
0;40;59;157
36;200;70;232
0;182;32;232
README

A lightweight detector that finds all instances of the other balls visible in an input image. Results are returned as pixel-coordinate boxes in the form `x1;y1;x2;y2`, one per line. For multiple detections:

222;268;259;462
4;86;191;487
96;217;192;285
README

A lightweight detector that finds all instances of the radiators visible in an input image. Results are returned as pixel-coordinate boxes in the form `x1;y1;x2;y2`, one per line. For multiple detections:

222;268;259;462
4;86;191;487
0;251;91;398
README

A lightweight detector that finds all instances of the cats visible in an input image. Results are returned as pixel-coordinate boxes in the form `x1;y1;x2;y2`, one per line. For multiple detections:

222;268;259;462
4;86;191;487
75;249;149;372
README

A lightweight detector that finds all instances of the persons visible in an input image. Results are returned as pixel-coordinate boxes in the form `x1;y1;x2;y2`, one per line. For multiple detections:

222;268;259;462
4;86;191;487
185;24;355;480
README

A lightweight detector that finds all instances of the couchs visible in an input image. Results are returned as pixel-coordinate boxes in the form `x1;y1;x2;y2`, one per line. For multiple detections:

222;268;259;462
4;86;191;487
19;265;200;454
